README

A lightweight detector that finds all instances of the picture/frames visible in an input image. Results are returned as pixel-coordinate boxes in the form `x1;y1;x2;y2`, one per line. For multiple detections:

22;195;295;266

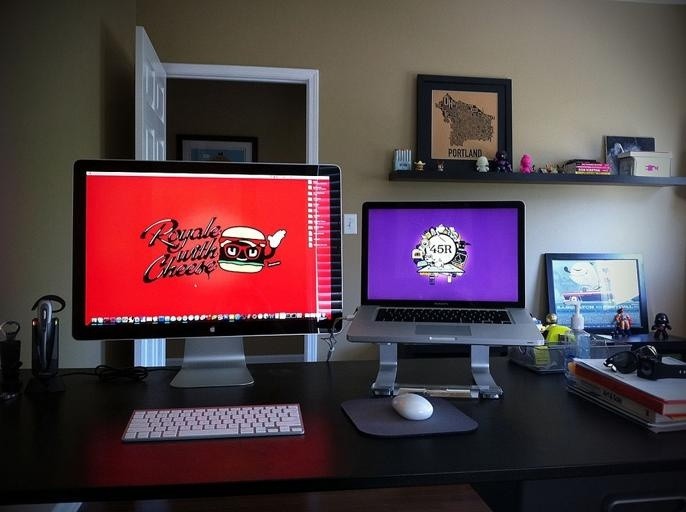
176;133;259;163
415;74;512;173
545;252;651;335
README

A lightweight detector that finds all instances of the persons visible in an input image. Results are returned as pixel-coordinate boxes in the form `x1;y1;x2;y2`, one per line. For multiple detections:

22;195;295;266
615;308;632;334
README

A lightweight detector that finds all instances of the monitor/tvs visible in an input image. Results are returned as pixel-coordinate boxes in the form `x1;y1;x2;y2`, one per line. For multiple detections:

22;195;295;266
72;158;344;388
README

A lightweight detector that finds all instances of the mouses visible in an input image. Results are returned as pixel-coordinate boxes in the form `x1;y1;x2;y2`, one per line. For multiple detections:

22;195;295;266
391;393;435;421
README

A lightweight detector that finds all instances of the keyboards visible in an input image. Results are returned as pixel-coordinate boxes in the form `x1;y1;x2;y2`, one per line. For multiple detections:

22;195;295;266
121;403;306;445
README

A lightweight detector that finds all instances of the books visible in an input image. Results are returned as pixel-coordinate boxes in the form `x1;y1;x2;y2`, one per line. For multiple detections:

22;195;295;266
564;357;686;435
564;158;611;175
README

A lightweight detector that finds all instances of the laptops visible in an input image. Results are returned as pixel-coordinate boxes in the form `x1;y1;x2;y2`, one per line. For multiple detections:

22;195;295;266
345;198;546;349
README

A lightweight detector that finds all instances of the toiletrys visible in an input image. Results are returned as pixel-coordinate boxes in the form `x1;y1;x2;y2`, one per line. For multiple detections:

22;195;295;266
563;299;592;377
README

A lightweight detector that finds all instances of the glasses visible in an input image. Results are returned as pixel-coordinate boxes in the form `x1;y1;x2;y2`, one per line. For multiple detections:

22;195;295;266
604;344;658;376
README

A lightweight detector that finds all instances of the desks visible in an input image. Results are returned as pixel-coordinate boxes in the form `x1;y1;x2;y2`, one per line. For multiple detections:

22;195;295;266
0;355;686;512
